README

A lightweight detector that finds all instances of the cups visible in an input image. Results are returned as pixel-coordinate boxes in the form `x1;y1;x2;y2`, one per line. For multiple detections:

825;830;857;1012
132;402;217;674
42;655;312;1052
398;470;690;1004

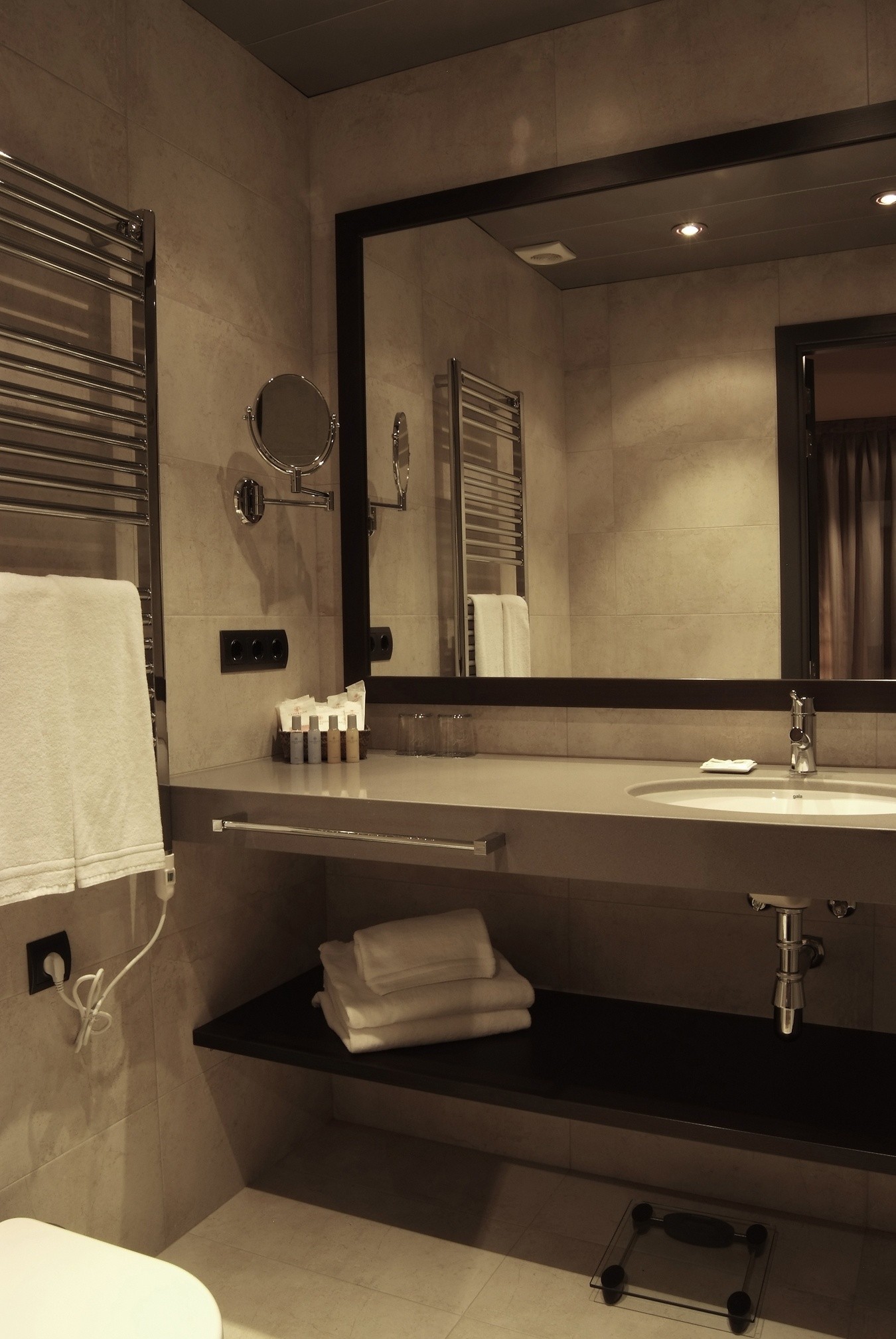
436;713;475;758
396;713;436;757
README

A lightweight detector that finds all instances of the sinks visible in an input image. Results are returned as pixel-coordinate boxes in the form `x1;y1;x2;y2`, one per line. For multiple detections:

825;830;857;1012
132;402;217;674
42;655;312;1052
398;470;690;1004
626;777;896;815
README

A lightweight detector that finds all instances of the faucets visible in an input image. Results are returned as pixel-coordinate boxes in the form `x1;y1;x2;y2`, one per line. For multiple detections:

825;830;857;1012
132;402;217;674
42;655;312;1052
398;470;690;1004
788;690;818;772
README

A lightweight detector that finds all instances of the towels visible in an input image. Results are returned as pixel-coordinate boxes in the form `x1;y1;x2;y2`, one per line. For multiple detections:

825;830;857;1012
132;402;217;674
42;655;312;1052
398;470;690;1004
308;911;539;1052
465;592;532;678
1;570;168;905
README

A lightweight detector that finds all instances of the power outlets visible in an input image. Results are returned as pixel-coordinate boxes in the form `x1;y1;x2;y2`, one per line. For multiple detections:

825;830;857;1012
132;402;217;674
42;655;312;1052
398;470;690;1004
369;626;393;663
26;931;72;995
219;629;290;675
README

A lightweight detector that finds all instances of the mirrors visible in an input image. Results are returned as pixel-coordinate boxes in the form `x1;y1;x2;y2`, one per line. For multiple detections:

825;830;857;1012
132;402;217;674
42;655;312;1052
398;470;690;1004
232;369;335;523
336;100;896;715
369;410;412;536
